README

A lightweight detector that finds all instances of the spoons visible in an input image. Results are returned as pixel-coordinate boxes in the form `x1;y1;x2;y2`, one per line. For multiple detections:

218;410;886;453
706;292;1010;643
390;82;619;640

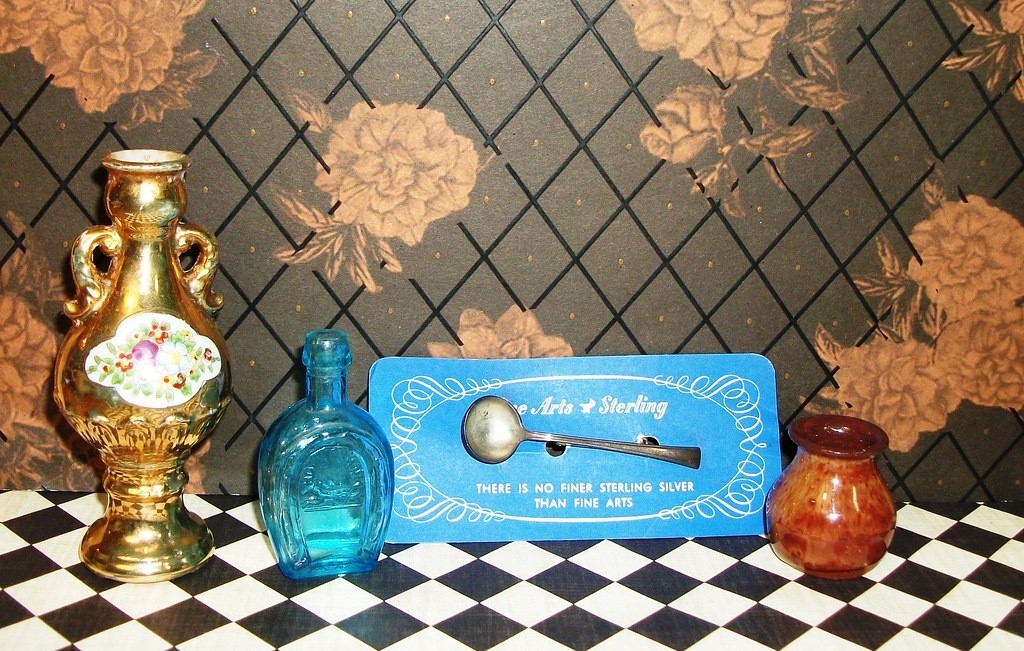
463;396;701;470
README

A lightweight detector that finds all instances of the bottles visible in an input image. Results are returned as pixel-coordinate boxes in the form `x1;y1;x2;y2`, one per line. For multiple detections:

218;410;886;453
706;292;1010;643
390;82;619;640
766;414;897;580
258;328;393;579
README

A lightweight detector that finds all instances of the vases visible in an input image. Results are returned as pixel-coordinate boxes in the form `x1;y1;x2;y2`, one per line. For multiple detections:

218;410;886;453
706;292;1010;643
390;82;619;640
53;147;235;585
766;414;897;579
